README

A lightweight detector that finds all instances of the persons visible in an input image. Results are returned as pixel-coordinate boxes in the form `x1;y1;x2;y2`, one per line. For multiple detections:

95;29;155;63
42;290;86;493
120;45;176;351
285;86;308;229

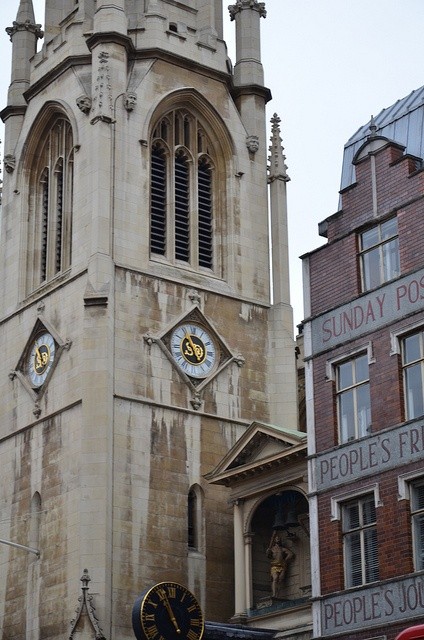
265;533;294;596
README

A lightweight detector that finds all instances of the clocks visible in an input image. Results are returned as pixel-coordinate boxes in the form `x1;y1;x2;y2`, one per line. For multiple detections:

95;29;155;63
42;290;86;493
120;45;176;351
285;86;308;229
23;327;57;391
133;581;206;640
167;319;222;380
